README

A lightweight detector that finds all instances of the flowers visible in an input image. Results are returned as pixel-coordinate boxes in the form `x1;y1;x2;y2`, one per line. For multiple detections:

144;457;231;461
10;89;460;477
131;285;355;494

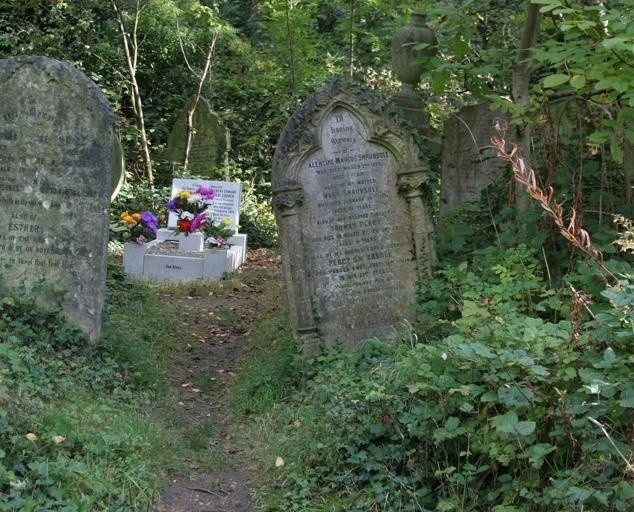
110;185;244;251
